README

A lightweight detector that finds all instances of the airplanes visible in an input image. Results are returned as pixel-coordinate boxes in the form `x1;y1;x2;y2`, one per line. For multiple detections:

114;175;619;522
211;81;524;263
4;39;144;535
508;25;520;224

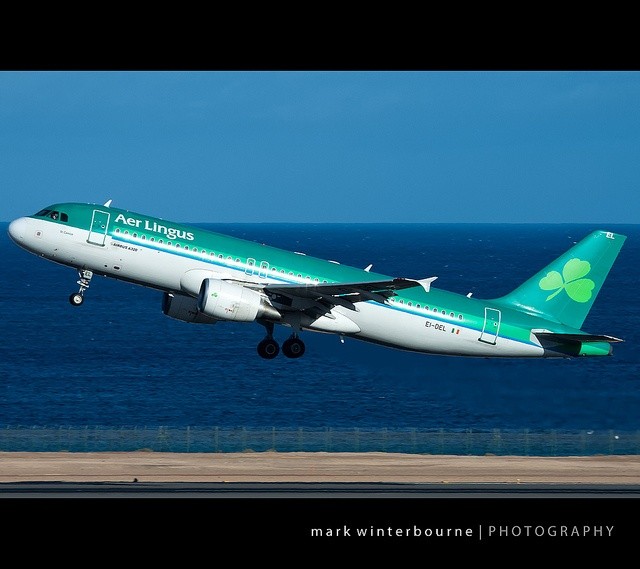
7;200;627;359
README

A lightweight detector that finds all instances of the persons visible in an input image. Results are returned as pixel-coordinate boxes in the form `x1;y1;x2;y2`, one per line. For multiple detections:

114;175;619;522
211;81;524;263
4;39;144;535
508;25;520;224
52;214;58;220
